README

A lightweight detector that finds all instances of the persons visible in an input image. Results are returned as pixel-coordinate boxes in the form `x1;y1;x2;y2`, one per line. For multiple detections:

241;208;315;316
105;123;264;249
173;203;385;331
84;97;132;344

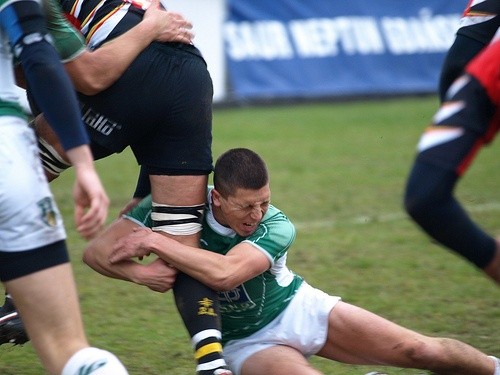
402;0;500;287
80;150;498;375
0;0;107;375
36;1;194;94
1;0;238;375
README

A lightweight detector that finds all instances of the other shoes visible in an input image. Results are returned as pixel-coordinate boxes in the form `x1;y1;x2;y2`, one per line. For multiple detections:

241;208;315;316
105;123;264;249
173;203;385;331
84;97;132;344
0;307;28;347
